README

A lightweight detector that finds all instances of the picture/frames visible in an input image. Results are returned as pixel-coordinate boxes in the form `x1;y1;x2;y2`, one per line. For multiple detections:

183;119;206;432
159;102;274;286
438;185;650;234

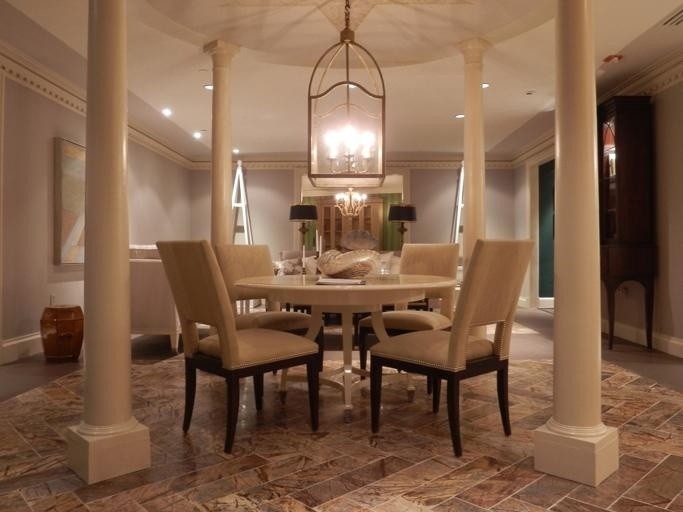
52;136;85;266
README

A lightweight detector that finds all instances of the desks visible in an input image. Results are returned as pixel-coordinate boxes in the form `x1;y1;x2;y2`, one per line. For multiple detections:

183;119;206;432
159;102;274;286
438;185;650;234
232;270;455;435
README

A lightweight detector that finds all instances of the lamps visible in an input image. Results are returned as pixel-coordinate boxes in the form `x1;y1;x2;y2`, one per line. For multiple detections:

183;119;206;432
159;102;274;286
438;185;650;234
306;1;387;187
334;187;367;218
288;204;317;250
387;202;416;252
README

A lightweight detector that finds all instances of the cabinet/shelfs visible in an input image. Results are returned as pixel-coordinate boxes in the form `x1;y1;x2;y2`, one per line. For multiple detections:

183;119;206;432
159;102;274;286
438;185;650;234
597;91;658;353
122;243;182;352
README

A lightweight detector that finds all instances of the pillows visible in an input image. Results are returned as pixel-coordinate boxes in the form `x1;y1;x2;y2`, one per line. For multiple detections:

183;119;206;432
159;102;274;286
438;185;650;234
269;255;302;277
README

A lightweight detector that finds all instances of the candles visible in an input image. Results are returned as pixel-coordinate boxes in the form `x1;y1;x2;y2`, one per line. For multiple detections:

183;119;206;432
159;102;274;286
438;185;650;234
301;244;305;267
314;229;319;251
318;236;321;256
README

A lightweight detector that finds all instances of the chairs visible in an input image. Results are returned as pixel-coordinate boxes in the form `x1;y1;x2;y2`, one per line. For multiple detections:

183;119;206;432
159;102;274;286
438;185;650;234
217;240;323;369
352;242;464;394
153;237;323;454
370;234;539;459
